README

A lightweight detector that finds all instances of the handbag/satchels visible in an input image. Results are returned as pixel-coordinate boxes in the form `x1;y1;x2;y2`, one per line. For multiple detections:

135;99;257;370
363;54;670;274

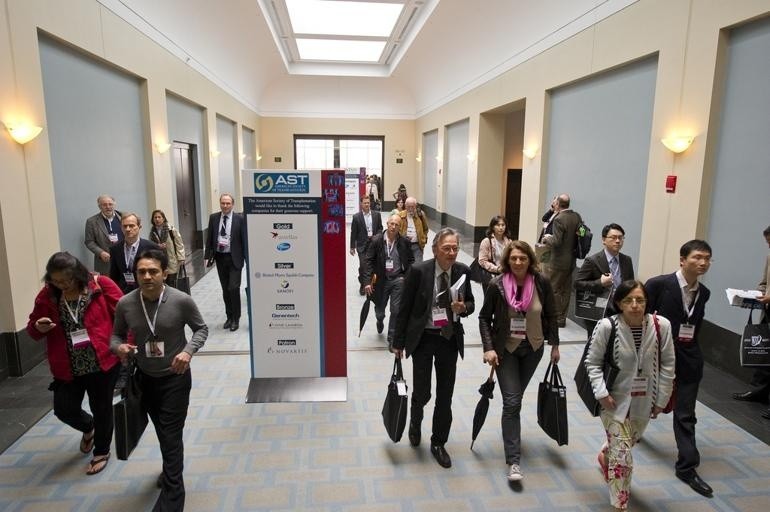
537;360;569;446
469;236;498;283
176;264;191;296
381;355;408;443
573;317;621;417
574;255;614;320
112;349;149;461
739;301;770;368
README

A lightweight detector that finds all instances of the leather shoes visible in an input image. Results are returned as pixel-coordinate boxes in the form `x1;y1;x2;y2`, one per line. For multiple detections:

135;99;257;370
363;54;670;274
759;408;770;419
430;442;452;469
408;419;422;447
675;467;713;497
360;284;366;296
229;319;239;331
156;470;167;488
732;389;768;402
376;317;385;334
223;318;233;329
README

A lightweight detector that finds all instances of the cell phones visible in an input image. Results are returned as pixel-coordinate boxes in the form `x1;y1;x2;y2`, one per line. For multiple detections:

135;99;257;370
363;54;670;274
37;320;50;325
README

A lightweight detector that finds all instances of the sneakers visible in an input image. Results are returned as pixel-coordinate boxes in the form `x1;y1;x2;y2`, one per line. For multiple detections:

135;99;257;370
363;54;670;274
507;463;524;482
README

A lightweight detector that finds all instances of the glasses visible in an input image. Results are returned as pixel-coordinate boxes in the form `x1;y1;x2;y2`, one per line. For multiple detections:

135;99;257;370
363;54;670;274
605;235;626;241
438;246;461;253
46;275;75;287
507;255;530;263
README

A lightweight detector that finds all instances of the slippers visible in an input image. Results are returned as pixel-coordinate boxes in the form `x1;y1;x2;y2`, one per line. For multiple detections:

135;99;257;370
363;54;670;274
86;452;110;475
79;433;94;454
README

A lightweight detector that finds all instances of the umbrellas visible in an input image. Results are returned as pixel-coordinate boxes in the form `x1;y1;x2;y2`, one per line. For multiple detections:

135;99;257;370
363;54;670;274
470;358;496;449
358;274;376;337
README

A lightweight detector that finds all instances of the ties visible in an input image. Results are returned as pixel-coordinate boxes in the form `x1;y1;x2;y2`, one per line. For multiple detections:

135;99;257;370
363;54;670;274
439;272;454;340
220;216;228;236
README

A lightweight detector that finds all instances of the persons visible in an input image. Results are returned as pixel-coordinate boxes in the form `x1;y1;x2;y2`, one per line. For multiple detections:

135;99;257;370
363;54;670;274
544;194;582;328
27;251;134;476
393;184;407;199
478;240;560;480
395;197;429;263
389;197;405;219
734;227;770;402
204;194;248;332
85;194;122;275
392;228;475;469
537;198;561;251
350;194;383;296
109;213;156;295
365;178;378;210
363;215;414;353
109;250;209;512
478;215;512;293
585;279;676;510
575;224;634;337
644;240;713;498
149;210;190;292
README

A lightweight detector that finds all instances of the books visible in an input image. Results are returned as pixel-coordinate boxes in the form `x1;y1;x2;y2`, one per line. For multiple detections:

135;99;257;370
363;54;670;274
450;273;465;322
726;288;768;310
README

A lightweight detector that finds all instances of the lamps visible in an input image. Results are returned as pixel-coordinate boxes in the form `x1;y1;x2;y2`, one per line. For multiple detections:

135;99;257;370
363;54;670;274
257;155;262;160
212;150;220;159
466;154;476;162
156;140;173;153
415;157;422;162
1;114;42;144
661;135;695;155
434;156;443;161
523;149;538;160
240;154;246;161
